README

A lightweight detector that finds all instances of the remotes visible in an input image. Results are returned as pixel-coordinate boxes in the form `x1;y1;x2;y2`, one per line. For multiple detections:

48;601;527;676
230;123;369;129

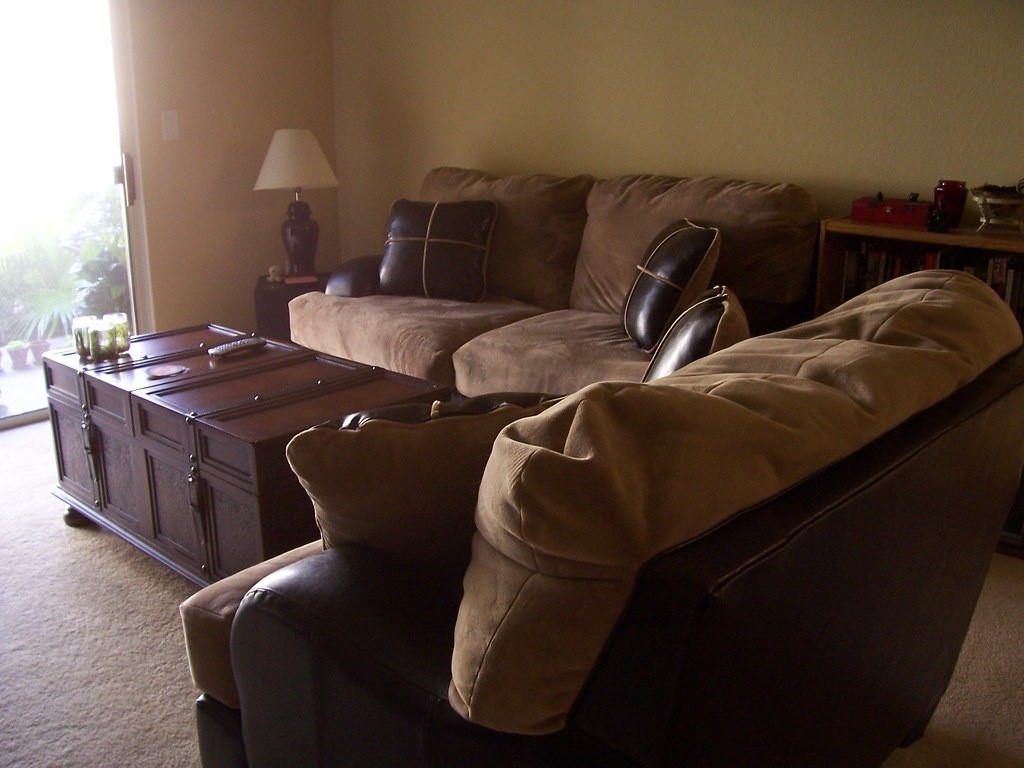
207;338;267;357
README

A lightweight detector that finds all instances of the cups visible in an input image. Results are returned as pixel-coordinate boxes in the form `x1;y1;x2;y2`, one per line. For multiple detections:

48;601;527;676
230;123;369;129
71;312;131;361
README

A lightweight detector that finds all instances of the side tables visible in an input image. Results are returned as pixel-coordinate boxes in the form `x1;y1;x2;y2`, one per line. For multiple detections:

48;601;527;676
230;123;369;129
251;274;329;341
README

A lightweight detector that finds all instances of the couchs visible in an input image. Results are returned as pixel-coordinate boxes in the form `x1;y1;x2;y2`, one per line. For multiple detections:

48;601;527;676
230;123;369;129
178;162;1023;768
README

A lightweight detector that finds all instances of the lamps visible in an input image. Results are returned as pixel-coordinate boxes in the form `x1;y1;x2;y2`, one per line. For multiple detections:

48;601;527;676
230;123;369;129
255;128;340;283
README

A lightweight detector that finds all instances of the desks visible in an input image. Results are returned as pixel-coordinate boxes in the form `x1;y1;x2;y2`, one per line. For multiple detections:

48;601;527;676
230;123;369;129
812;217;1024;326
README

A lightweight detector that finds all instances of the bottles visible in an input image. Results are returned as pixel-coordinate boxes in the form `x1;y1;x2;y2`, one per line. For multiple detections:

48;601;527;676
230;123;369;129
933;179;969;228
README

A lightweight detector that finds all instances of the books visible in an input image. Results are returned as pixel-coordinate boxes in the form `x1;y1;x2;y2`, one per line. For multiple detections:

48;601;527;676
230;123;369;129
1001;502;1024;538
841;240;1024;327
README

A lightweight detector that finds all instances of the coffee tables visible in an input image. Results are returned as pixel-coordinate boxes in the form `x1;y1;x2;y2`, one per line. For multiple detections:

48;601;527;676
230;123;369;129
40;321;458;593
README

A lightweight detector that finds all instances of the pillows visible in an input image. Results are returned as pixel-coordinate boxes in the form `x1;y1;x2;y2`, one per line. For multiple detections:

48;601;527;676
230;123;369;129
377;198;499;304
447;267;1024;736
620;218;720;353
640;287;752;385
284;385;568;553
